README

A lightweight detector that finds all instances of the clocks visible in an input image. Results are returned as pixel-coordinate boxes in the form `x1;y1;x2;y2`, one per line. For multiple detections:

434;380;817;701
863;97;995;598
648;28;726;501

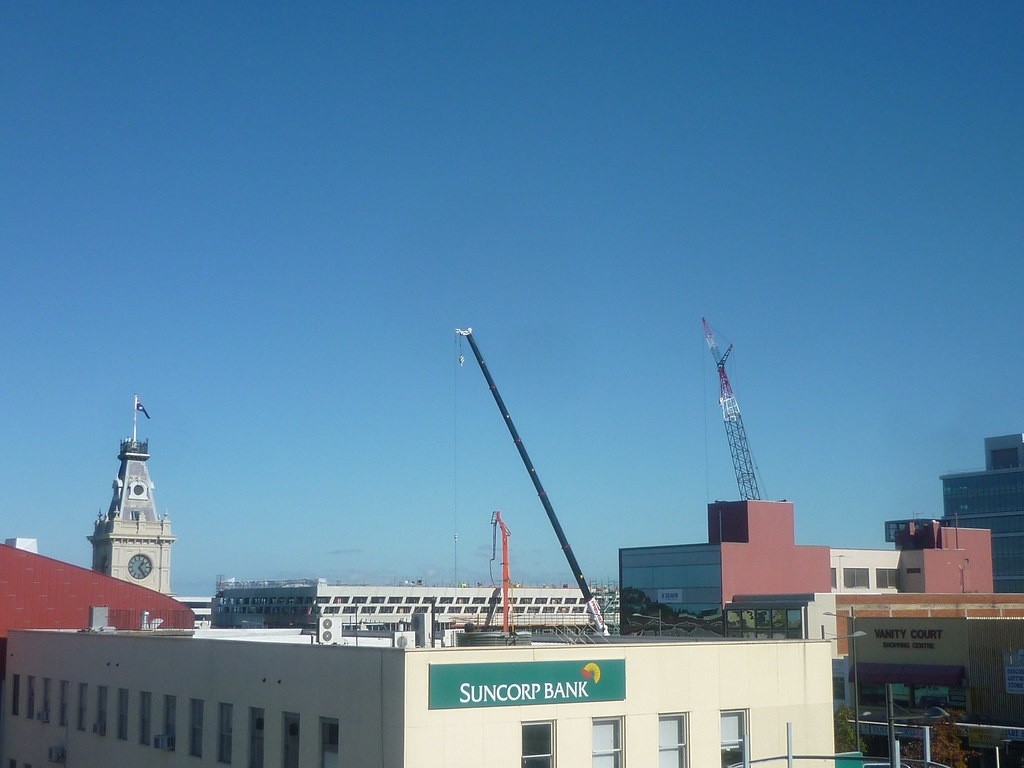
128;554;152;580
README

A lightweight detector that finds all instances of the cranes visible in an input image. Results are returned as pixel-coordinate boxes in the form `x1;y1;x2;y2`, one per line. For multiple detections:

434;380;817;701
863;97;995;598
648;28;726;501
454;327;608;635
488;510;511;633
701;316;761;501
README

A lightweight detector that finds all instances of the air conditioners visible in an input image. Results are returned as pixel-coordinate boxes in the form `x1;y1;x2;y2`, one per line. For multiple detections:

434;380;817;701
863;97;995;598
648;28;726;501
393;630;416;650
48;746;65;763
316;615;343;645
154;734;174;752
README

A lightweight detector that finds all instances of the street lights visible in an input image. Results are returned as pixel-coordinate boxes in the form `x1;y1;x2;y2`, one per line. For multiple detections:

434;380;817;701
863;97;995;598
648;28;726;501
823;606;860;752
